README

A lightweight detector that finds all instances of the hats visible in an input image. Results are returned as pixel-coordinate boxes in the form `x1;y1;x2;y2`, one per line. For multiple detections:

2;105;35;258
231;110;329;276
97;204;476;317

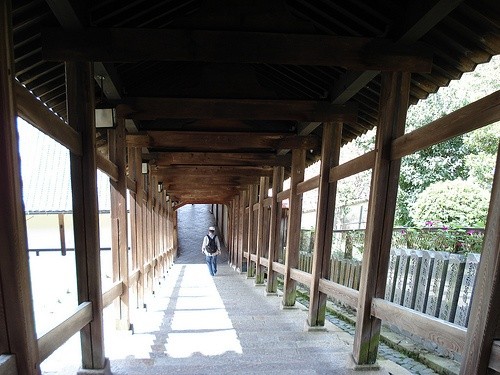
208;226;215;231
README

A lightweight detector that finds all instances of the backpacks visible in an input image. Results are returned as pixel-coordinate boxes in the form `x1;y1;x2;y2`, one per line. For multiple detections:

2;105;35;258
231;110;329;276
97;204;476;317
206;235;216;253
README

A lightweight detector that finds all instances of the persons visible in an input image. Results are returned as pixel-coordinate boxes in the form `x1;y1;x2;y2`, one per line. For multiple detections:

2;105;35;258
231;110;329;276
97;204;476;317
201;226;222;277
